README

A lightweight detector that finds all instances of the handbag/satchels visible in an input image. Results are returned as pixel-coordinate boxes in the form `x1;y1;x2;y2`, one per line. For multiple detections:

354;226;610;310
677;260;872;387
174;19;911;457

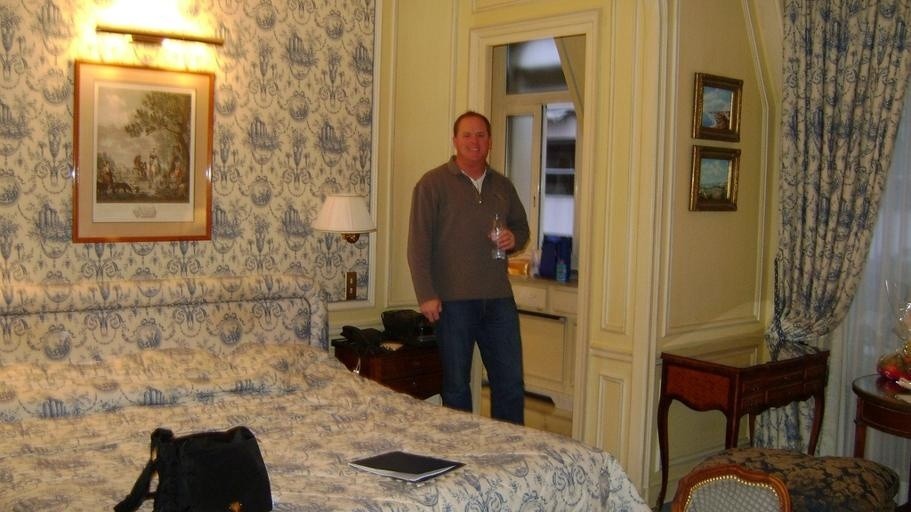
111;427;271;512
382;309;426;342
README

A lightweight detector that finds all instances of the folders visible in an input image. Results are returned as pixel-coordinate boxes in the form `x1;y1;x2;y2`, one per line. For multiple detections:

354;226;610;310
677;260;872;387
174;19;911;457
345;447;465;485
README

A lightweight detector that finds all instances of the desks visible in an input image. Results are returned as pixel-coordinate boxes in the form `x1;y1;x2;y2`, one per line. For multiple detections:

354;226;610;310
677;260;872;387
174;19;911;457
651;335;829;511
852;373;911;512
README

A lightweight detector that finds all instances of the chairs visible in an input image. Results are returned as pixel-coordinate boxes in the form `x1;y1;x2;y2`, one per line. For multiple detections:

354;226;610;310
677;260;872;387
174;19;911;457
672;464;791;512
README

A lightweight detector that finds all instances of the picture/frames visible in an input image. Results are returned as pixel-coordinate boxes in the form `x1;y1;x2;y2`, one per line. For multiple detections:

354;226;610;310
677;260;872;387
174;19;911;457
692;71;744;142
73;59;215;243
689;146;742;211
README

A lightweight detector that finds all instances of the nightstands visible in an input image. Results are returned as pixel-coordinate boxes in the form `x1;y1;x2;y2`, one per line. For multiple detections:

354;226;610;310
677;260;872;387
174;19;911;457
335;332;445;403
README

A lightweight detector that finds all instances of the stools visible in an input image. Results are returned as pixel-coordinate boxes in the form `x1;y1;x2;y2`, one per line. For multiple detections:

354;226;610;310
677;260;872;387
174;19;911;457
692;447;900;511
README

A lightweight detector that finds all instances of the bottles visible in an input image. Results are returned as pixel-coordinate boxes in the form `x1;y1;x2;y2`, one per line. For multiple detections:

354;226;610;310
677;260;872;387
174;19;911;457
556;259;567;282
490;213;506;259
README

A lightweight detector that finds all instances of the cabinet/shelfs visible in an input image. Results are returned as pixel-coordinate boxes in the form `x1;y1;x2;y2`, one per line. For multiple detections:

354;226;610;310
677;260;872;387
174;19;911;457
481;278;578;418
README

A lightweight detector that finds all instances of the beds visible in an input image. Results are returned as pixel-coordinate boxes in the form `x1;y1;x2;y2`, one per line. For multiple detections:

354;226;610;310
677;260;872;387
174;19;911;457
0;275;622;512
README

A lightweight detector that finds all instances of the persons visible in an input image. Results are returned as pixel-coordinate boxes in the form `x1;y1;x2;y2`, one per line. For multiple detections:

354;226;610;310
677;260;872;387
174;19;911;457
407;112;530;428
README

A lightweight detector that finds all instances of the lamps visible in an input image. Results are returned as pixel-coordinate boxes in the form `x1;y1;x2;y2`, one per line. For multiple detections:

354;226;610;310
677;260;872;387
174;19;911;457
310;193;377;243
